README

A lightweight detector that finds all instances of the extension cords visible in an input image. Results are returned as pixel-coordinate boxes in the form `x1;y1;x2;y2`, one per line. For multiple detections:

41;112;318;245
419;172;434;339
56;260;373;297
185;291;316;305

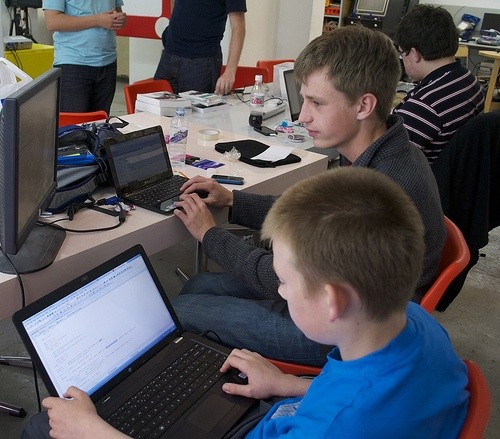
251;100;286;121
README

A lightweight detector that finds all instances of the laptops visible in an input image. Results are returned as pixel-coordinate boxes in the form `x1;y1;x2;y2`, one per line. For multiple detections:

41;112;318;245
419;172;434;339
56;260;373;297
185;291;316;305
11;243;257;439
104;125;208;216
282;68;304;128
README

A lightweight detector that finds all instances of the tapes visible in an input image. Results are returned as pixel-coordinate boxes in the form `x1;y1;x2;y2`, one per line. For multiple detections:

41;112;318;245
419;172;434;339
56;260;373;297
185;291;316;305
197;129;220;140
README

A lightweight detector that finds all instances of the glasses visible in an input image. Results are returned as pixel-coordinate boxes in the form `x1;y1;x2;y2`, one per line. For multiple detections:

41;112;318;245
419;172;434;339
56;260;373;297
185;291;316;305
398;49;407;59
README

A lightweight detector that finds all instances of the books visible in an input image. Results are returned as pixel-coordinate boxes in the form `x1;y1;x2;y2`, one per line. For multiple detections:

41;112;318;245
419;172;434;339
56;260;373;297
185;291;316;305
136;91;227;116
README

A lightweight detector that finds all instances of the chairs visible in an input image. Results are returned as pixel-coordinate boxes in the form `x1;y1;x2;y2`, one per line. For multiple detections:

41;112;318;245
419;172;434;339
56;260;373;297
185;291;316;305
57;60;500;439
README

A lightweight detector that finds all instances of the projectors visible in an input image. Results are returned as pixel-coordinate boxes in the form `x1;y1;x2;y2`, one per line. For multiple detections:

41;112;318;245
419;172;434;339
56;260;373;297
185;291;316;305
3;36;32;51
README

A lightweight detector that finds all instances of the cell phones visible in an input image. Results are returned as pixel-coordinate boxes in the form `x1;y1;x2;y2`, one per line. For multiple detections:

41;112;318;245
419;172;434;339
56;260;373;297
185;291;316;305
254;125;277;136
211;175;244;185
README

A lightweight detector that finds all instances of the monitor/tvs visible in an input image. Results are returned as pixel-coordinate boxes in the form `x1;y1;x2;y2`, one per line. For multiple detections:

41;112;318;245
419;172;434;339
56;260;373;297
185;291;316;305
1;68;66;274
356;0;387;13
480;13;500;37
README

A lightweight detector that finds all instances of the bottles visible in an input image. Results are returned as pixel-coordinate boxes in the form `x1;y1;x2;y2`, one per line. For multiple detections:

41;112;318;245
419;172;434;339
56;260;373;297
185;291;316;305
249;74;265;127
168;106;189;171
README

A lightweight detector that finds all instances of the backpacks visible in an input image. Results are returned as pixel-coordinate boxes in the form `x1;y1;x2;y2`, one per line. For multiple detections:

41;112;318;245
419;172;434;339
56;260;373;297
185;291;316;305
43;116;129;210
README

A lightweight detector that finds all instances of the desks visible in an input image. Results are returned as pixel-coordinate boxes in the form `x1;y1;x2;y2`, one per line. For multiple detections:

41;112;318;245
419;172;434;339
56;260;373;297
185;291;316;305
0;81;338;321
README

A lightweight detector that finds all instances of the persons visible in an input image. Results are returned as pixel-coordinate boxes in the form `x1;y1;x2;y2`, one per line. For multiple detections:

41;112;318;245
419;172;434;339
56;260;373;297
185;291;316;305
42;0;126;117
392;4;486;165
22;166;470;439
153;0;248;94
171;25;446;368
